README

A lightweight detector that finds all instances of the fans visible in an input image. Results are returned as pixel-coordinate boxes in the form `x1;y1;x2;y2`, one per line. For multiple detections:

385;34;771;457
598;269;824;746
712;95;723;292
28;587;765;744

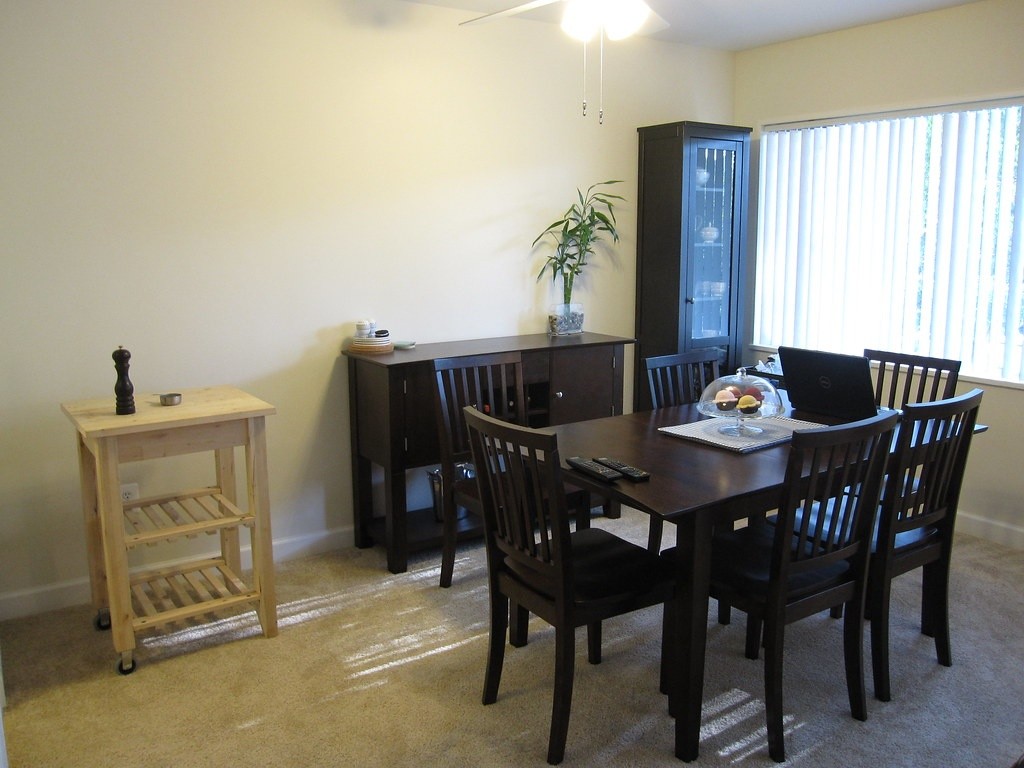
458;0;671;40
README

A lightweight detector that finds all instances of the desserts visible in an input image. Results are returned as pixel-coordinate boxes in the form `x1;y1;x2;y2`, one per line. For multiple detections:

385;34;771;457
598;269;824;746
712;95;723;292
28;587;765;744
712;385;764;414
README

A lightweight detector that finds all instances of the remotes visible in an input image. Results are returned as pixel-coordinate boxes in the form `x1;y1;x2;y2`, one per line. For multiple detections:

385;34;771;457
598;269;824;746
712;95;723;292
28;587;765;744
565;456;622;482
591;456;650;481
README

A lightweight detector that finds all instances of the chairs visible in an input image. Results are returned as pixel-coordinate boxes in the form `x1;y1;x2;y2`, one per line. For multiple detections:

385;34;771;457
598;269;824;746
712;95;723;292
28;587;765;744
668;408;899;764
796;348;962;511
641;347;766;625
761;385;986;704
432;350;589;589
464;404;676;766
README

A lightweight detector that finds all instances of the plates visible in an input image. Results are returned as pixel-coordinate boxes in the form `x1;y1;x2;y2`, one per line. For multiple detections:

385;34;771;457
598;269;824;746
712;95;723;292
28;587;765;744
352;336;392;346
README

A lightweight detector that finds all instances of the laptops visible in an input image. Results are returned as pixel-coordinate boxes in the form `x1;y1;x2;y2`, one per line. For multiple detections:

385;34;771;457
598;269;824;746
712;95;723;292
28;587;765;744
777;346;903;421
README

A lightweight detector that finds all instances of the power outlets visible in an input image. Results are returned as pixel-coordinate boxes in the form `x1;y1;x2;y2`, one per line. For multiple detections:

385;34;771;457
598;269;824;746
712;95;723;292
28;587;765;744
120;483;141;515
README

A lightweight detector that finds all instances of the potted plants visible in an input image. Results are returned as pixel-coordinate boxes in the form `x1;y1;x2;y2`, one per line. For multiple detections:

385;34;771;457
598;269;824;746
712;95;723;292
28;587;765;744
530;180;629;338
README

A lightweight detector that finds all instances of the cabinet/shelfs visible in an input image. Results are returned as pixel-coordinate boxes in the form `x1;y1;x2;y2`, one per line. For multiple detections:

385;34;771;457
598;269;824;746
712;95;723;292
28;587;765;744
633;120;753;415
342;330;635;575
62;381;278;674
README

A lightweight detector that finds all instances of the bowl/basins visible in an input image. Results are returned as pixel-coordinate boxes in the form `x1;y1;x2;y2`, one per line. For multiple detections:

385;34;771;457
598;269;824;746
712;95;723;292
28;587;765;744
710;282;724;297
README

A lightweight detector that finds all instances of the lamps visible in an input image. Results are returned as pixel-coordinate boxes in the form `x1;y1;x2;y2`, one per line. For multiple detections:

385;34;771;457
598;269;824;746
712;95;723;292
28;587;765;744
560;0;651;125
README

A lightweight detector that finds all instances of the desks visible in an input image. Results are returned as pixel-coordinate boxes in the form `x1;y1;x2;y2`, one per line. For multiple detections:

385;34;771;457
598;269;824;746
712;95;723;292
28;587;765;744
467;389;989;763
